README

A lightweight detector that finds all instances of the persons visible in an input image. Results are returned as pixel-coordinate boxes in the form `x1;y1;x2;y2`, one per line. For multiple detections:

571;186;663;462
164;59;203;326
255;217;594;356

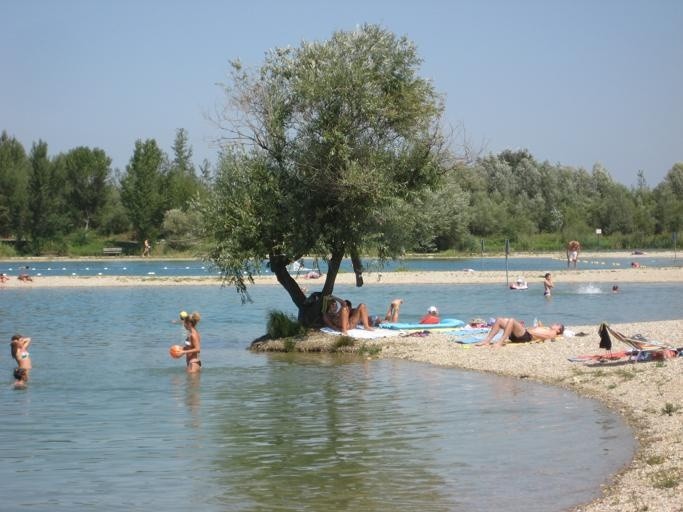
631;261;640;268
475;317;564;348
339;298;404;325
17;273;31;281
174;312;202;374
566;241;581;270
11;368;29;388
170;310;187;325
417;306;439;324
321;294;374;337
8;334;33;372
139;238;150;257
510;277;529;290
0;272;9;284
612;285;618;293
542;273;552;297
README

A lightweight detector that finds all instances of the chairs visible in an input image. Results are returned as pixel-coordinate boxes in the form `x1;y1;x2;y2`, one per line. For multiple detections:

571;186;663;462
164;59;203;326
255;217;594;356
602;320;674;371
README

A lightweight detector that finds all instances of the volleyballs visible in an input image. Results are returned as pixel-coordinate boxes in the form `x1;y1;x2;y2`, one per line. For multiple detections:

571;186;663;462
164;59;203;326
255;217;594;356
170;345;185;358
179;311;188;320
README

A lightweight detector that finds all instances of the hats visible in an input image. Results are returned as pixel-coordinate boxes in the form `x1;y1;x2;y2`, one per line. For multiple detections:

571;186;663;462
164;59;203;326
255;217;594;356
427;306;435;313
